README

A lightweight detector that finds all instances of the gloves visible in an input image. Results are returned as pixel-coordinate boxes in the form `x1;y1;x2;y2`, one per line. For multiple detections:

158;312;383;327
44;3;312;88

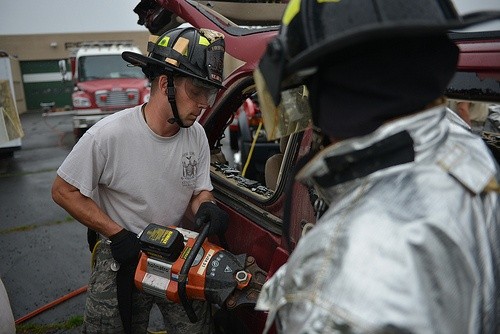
109;227;142;267
195;200;227;233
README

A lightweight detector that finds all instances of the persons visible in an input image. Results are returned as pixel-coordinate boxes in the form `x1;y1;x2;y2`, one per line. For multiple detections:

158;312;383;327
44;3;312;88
51;23;226;334
253;0;500;334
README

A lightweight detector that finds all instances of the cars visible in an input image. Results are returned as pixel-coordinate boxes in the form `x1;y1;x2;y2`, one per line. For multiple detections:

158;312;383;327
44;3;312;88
129;0;500;333
58;43;154;142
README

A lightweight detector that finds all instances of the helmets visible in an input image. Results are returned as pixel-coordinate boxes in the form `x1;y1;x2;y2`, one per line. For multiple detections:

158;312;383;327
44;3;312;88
121;27;229;91
278;0;464;87
